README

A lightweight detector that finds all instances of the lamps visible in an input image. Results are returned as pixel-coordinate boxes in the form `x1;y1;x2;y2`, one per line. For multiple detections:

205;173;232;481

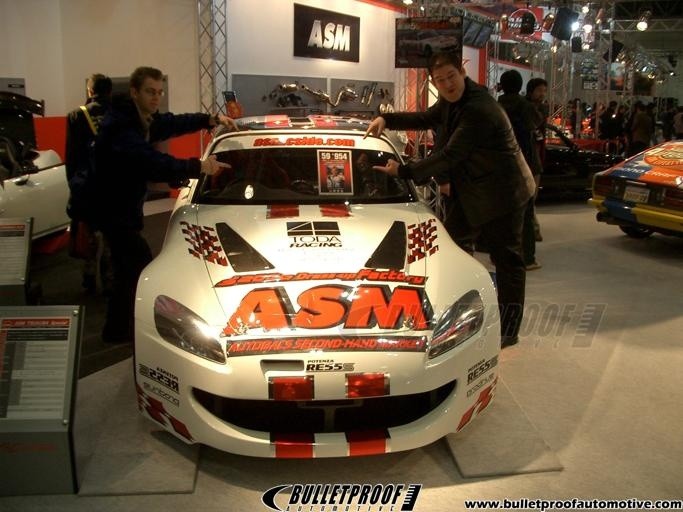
550;36;561;54
635;11;652;33
511;47;521;61
499;1;554;36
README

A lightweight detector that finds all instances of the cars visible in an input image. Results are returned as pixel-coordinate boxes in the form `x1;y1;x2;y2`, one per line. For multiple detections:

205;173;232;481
131;111;501;462
588;139;682;243
0;88;71;244
405;102;630;203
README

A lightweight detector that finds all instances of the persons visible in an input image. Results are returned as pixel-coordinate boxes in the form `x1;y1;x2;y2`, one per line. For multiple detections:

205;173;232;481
90;67;239;344
363;51;537;349
524;78;560;241
567;96;683;157
498;69;544;270
65;73;113;288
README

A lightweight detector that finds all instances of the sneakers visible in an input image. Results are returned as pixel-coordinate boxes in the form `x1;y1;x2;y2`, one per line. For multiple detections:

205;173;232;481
526;260;542;270
535;231;543;241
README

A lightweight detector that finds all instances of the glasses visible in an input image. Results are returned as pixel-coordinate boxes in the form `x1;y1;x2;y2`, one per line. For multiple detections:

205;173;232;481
141;88;165;97
